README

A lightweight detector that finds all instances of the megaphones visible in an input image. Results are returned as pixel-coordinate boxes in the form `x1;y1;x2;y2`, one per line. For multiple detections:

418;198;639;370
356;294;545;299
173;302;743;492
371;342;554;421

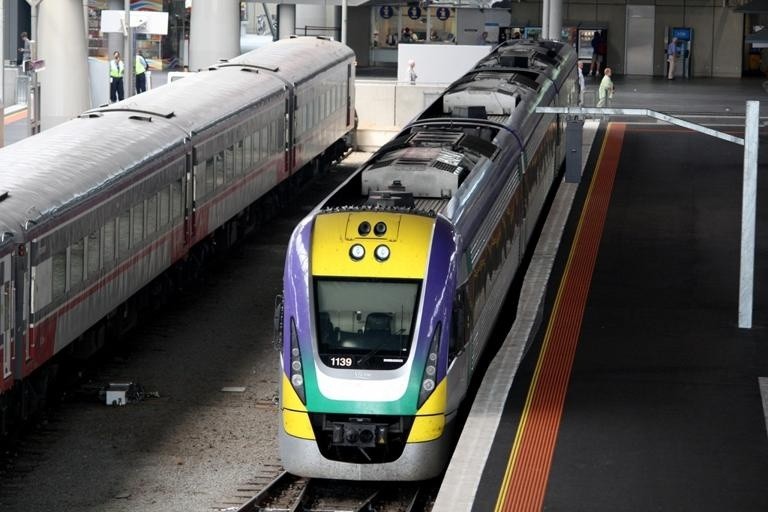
33;58;46;73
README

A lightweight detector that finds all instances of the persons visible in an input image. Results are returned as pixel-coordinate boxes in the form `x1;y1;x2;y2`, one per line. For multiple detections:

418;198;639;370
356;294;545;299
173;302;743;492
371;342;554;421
108;51;124;102
480;32;488;45
408;60;418;85
586;32;605;77
135;50;148;94
386;27;440;46
667;36;678;80
596;67;615;107
578;61;586;107
18;32;31;65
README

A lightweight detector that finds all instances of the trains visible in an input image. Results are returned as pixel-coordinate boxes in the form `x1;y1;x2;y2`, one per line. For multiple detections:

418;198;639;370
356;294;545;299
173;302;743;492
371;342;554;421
0;36;360;399
277;33;584;482
